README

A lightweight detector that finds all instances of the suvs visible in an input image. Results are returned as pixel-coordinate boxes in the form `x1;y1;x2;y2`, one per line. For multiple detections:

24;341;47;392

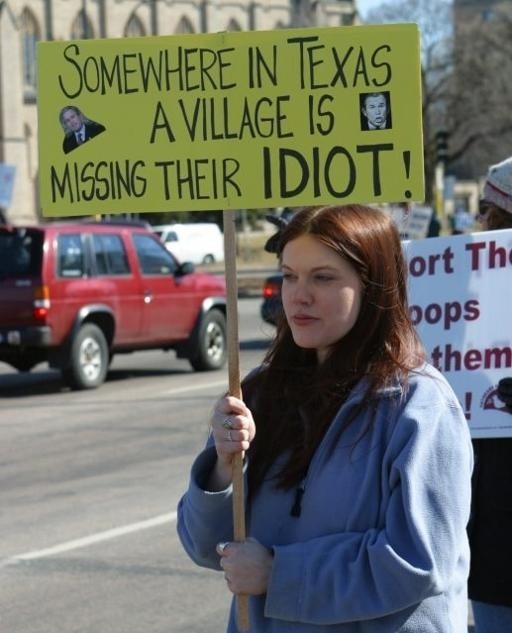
1;217;227;390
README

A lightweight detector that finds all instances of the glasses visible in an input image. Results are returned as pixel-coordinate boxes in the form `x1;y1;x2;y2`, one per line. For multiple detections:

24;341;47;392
479;199;494;215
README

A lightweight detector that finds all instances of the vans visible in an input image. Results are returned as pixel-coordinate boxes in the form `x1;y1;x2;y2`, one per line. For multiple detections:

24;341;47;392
156;221;223;266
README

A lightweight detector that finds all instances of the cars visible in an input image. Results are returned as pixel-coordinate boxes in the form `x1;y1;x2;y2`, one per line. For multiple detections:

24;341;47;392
261;275;285;320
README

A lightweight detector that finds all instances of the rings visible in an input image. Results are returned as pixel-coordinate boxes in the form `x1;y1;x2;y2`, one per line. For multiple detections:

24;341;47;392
226;430;232;441
223;414;234;429
219;540;230;553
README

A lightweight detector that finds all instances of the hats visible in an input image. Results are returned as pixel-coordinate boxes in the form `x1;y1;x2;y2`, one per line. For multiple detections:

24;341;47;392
482;156;512;214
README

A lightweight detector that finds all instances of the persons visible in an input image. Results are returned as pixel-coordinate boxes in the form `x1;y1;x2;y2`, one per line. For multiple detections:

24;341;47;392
361;91;391;130
473;156;511;230
426;208;442;238
173;203;479;631
447;205;476;234
61;105;107;153
